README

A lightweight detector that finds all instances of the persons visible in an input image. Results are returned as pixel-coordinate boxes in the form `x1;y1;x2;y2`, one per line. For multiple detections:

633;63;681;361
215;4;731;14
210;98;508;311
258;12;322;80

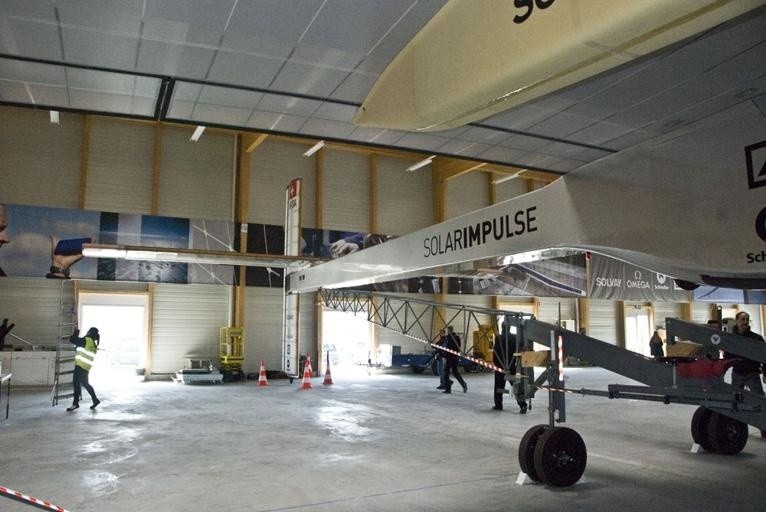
491;321;528;413
650;331;664;356
722;311;766;440
440;325;467;394
65;326;100;411
0;203;11;277
430;328;454;389
45;233;96;279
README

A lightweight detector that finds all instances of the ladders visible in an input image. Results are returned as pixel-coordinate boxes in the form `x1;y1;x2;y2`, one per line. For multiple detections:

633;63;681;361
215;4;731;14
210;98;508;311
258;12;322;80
52;279;80;405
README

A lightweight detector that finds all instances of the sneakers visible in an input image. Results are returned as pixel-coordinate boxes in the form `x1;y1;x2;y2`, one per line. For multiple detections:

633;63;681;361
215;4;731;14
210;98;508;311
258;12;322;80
67;404;79;410
90;399;100;409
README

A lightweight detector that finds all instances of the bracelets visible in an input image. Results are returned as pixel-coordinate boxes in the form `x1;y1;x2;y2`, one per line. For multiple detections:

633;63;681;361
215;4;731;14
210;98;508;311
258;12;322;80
48;264;71;277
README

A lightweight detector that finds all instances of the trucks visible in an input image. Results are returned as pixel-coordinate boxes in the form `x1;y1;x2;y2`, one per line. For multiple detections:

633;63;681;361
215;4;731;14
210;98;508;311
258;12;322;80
393;323;495;374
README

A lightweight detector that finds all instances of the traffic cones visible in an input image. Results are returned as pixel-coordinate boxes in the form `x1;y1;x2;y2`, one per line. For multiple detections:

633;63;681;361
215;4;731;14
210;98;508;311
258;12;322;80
302;352;314;388
323;365;333;384
259;359;268;385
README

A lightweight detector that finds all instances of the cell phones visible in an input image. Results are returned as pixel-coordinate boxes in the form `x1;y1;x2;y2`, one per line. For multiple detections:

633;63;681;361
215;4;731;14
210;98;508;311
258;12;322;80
54;238;91;255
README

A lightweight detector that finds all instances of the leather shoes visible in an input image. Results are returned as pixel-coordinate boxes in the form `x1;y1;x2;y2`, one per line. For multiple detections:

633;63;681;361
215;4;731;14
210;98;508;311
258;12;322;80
520;406;527;414
492;405;502;410
437;383;451;393
463;386;467;393
